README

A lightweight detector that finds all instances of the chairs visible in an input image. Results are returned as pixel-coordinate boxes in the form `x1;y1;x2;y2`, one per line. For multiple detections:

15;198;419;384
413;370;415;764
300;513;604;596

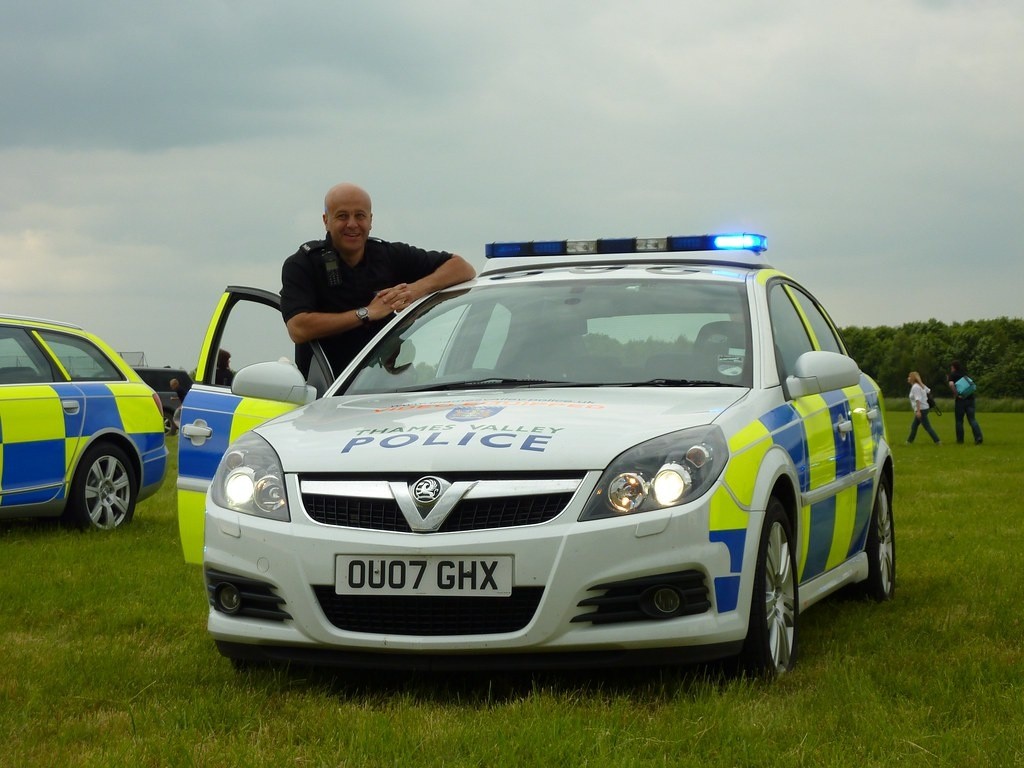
690;320;788;386
569;356;632;382
0;367;39;384
503;342;574;380
643;352;691;382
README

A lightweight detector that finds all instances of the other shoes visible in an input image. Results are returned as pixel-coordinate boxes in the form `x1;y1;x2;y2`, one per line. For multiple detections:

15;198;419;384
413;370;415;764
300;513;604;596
904;441;911;445
933;441;941;445
975;436;983;445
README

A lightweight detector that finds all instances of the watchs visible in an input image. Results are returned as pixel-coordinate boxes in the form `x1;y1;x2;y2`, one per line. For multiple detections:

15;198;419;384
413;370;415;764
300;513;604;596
356;306;370;323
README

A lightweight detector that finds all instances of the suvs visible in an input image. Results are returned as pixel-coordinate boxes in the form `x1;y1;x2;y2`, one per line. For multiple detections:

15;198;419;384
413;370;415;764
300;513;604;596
92;367;194;437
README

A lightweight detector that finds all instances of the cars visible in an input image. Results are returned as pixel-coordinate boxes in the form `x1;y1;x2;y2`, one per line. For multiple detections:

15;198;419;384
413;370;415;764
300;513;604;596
173;231;895;680
0;313;170;532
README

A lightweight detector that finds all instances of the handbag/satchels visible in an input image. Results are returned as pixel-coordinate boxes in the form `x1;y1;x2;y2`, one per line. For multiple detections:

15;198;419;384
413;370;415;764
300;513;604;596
927;392;942;417
954;376;977;399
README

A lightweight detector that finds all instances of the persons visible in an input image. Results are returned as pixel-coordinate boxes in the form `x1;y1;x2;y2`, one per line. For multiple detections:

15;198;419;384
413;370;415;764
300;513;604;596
279;184;477;381
905;371;940;444
170;379;189;403
215;349;234;386
947;361;983;444
